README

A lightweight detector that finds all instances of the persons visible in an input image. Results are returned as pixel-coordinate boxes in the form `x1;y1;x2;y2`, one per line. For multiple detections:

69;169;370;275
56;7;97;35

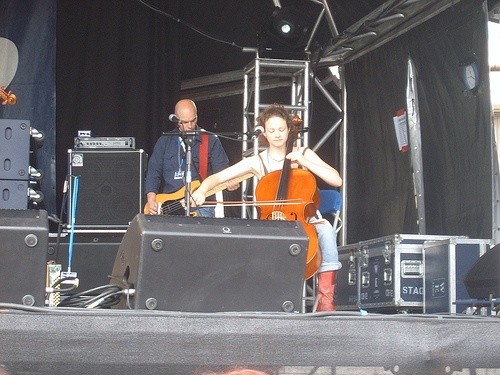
98;98;240;308
190;106;342;312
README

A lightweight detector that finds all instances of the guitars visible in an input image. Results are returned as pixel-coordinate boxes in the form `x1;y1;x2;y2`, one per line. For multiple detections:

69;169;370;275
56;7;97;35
143;172;253;218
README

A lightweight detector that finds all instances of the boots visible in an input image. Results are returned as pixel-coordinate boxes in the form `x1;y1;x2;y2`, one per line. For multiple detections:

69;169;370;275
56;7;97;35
316;272;337;311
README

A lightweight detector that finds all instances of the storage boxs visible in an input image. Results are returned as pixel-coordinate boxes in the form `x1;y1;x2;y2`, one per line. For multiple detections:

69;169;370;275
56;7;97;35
359;234;468;313
333;242;360;310
422;238;496;315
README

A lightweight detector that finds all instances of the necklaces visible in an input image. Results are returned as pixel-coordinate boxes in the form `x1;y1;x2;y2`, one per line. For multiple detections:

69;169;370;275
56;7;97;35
268;150;285;163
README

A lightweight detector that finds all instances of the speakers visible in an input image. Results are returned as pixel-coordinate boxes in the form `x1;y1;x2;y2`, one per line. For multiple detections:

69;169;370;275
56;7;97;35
1;180;28;210
465;242;500;298
68;147;146;233
0;119;29;179
0;209;49;307
112;215;309;313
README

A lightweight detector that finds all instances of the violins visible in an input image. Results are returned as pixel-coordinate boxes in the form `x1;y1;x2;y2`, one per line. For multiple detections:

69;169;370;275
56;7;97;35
0;82;18;107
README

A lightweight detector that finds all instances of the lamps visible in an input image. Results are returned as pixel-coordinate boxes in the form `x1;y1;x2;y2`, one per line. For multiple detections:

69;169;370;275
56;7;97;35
271;6;311;48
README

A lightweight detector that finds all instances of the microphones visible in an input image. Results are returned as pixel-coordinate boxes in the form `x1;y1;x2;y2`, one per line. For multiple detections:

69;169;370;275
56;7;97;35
169;113;183;124
250;126;265;142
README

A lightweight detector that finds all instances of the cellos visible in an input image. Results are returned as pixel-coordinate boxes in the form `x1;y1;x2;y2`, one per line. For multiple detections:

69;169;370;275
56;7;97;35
253;114;323;282
